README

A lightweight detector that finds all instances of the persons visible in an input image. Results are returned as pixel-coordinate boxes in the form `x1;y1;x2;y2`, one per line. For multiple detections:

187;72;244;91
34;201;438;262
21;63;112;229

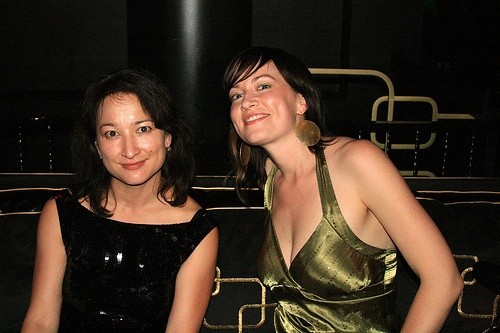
222;45;464;333
20;69;219;333
